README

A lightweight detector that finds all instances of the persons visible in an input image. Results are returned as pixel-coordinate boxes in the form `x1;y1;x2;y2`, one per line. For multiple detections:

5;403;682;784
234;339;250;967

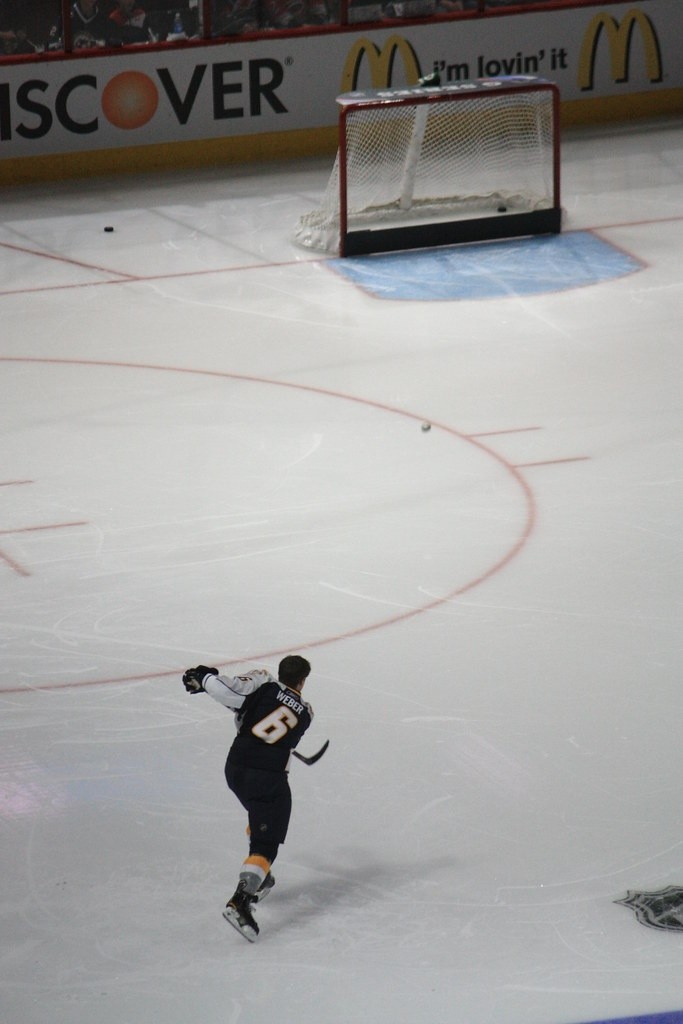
182;656;311;941
0;0;481;58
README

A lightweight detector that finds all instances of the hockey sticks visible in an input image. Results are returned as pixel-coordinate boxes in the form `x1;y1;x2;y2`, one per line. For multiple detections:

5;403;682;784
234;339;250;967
188;675;330;766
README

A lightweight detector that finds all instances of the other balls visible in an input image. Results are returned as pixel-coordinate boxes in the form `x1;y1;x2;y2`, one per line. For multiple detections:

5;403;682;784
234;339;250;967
104;226;113;231
498;207;506;212
421;424;431;431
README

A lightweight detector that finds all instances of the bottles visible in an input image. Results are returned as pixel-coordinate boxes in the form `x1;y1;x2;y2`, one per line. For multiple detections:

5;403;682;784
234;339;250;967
173;12;183;33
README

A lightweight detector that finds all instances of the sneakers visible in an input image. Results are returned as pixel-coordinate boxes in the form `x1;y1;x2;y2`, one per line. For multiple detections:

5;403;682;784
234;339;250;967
246;870;275;904
224;891;260;944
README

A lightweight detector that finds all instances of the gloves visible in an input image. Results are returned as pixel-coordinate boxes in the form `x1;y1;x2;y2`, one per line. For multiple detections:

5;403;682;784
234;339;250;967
182;665;219;695
